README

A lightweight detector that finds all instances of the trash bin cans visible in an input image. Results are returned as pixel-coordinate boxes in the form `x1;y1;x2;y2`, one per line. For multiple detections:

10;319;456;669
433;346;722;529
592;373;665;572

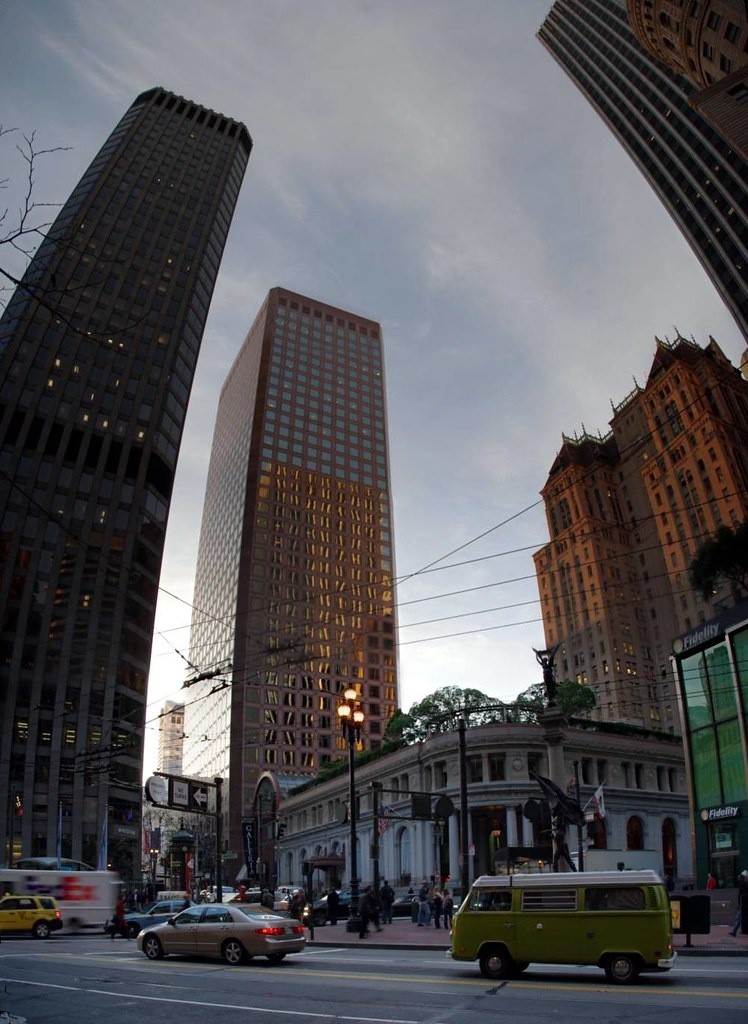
411;896;420;922
666;891;712;948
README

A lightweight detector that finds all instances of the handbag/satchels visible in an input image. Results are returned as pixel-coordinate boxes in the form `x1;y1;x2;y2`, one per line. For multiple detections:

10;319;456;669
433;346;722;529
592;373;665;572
111;914;116;923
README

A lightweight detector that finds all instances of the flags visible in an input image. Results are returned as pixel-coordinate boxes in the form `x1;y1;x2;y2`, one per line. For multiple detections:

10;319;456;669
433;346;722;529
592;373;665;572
593;785;606;819
98;819;106;870
378;803;390;836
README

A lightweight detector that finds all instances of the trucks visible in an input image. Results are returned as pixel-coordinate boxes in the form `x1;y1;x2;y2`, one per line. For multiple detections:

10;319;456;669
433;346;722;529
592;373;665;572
0;870;123;932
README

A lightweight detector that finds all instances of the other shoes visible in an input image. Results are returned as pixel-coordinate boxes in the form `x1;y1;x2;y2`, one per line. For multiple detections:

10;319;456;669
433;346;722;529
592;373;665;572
360;935;366;938
417;923;423;926
365;929;370;933
377;928;383;932
730;933;735;937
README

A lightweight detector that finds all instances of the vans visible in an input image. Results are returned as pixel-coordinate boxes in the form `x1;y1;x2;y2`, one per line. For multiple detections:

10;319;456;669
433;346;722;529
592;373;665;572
449;868;679;983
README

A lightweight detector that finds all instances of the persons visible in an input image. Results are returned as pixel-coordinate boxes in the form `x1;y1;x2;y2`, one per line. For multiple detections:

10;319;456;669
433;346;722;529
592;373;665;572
408;887;414;894
178;895;190;913
291;887;306;922
551;807;578;872
417;881;453;928
706;872;717;890
533;645;561;703
262;888;274;910
126;889;145;910
728;868;748;937
487;892;511;911
327;887;339;925
358;880;395;939
665;872;675;892
110;894;131;941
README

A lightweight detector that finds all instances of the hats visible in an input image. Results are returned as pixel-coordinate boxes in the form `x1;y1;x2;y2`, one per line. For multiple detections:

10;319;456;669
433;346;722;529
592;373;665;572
423;881;428;884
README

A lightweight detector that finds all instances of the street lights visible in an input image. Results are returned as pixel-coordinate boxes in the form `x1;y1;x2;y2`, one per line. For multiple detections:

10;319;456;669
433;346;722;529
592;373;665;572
337;686;368;933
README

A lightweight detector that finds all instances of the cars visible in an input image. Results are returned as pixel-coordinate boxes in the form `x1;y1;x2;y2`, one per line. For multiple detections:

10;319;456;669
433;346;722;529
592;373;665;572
113;901;199;938
0;894;65;939
200;884;436;918
303;888;370;925
136;902;308;965
